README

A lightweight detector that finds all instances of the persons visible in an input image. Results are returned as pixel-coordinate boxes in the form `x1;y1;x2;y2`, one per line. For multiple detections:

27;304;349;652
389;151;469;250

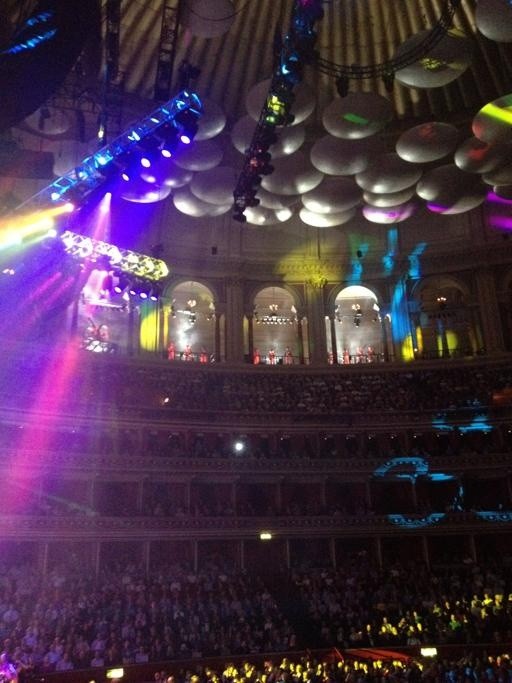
0;344;511;682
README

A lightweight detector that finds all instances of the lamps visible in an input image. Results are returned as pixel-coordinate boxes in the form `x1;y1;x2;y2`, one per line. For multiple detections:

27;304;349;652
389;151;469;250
0;0;397;328
